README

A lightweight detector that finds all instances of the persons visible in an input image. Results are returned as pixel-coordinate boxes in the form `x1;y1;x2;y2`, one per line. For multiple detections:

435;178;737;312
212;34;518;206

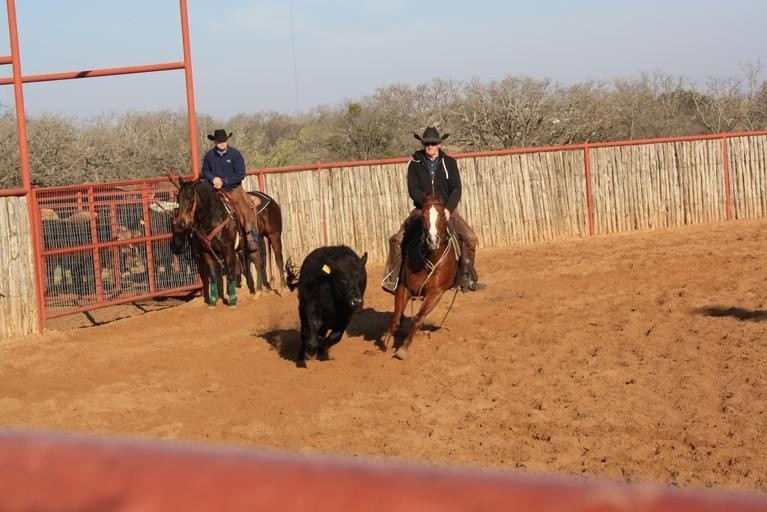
381;124;487;293
200;127;260;255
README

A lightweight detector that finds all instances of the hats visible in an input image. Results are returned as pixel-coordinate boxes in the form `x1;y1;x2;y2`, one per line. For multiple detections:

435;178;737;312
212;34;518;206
208;129;233;140
414;126;450;145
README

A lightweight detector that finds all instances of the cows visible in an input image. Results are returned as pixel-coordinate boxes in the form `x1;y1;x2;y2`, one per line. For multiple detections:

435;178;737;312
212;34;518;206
295;243;369;368
42;215;142;295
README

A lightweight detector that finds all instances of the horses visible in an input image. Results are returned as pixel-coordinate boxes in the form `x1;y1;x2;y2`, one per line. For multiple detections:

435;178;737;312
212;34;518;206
172;174;290;310
376;192;462;360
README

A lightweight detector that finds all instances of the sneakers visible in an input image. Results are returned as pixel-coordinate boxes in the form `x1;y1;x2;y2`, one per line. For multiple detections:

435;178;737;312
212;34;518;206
465;280;487;291
245;239;260;251
